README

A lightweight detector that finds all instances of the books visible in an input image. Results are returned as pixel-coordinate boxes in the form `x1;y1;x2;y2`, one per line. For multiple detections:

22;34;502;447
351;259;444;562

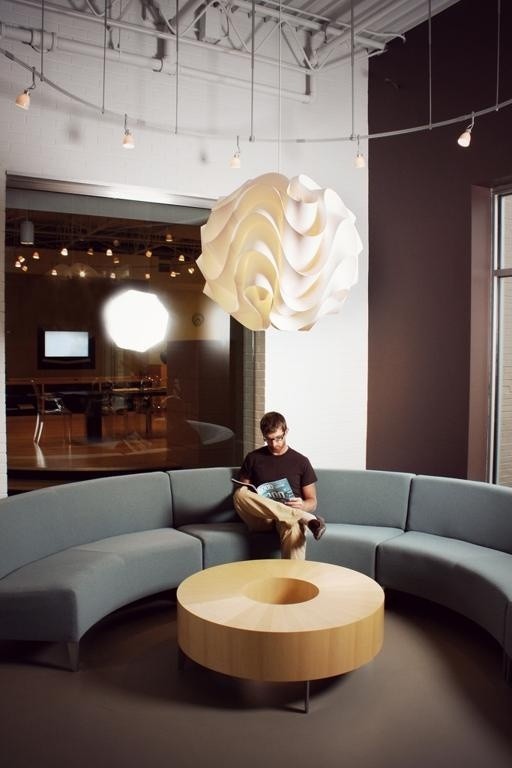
231;478;295;503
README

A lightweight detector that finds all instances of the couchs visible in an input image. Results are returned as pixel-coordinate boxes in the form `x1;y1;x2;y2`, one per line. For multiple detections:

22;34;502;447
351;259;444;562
0;465;512;672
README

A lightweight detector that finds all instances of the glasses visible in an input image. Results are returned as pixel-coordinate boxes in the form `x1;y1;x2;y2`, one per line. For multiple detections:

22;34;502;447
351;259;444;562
263;432;285;441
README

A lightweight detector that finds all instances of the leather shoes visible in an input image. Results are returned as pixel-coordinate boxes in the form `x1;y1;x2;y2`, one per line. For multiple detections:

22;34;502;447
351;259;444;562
308;514;326;540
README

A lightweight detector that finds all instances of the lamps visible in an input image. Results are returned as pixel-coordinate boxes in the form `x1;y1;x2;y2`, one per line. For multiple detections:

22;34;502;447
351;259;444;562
457;113;475;149
20;209;34;245
196;1;364;334
16;68;36;110
123;115;135;149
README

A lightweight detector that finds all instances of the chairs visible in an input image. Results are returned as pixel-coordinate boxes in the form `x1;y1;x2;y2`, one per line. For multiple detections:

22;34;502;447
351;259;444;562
30;376;167;444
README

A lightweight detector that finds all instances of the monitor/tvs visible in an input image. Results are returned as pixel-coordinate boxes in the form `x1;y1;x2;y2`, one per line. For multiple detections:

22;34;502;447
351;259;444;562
44;330;89;358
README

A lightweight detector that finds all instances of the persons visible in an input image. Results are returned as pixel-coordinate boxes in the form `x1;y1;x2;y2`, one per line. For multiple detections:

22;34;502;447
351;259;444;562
233;412;326;560
